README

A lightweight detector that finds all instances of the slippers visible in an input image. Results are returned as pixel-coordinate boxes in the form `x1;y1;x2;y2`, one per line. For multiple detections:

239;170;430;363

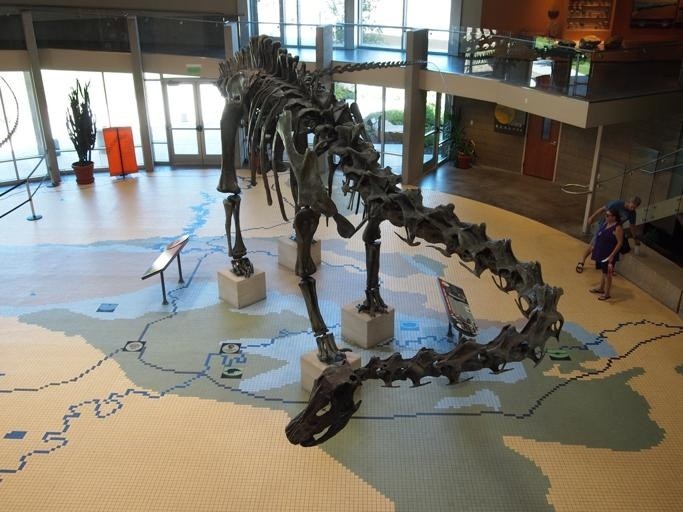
589;288;611;301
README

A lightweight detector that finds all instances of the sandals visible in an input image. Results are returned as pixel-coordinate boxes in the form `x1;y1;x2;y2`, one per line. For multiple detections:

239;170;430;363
576;262;584;274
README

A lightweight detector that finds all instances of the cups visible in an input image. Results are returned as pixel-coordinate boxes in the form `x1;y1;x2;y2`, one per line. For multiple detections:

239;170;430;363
634;242;641;256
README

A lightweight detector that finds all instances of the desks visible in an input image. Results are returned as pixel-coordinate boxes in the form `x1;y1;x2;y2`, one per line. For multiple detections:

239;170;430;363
486;48;539;81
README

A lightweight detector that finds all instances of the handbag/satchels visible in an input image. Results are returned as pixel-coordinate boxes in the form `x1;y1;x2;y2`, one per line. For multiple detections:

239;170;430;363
619;238;631;255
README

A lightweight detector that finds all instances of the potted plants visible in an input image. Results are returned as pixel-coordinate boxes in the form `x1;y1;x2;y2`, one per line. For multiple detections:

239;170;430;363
63;75;99;185
438;121;479;168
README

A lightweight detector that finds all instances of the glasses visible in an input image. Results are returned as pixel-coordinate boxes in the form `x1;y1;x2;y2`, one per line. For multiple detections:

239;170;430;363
606;214;613;217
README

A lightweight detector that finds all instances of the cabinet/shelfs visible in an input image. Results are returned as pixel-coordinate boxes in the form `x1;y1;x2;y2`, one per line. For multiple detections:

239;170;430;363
561;0;616;33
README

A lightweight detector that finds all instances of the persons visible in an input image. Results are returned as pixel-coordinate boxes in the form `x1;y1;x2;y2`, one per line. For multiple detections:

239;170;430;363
576;195;642;300
